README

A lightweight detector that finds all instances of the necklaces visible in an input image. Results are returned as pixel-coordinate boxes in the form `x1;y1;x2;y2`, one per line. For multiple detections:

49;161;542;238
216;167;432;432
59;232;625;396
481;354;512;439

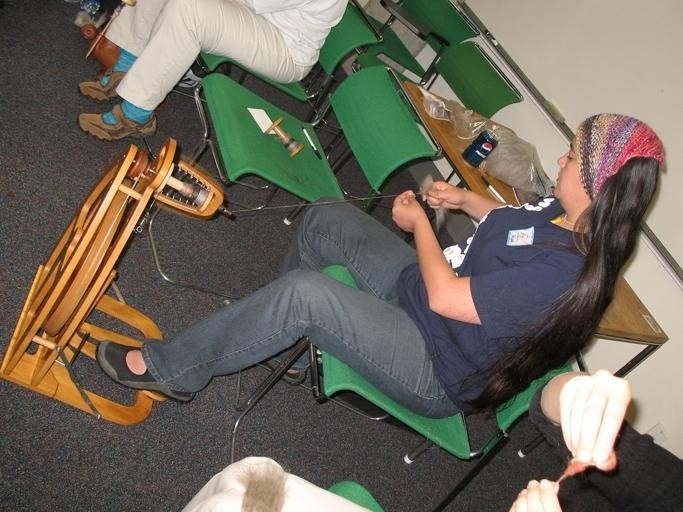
562;212;588;227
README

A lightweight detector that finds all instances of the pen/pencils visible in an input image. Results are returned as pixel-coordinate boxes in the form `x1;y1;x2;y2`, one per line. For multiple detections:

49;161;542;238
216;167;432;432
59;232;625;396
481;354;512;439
482;176;507;204
302;126;323;159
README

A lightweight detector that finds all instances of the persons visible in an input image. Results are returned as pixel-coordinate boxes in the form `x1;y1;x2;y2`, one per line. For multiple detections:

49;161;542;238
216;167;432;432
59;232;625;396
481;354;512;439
96;114;665;406
507;371;683;511
76;0;352;141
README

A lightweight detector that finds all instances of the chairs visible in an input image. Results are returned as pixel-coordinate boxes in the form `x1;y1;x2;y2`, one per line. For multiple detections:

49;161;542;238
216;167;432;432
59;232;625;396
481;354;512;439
149;62;445;301
307;0;480;128
230;265;591;512
316;41;523;211
141;0;384;190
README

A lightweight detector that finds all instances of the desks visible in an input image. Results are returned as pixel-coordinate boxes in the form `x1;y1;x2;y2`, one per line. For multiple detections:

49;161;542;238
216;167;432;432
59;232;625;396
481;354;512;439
282;81;670;382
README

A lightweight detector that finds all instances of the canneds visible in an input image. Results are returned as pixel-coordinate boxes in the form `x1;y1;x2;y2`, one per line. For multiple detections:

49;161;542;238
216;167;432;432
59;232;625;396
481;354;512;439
457;129;500;165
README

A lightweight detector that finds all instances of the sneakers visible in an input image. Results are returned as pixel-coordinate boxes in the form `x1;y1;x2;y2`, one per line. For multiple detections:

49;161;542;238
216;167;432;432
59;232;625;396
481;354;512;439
95;340;197;403
76;103;157;146
258;359;308;386
77;64;127;105
